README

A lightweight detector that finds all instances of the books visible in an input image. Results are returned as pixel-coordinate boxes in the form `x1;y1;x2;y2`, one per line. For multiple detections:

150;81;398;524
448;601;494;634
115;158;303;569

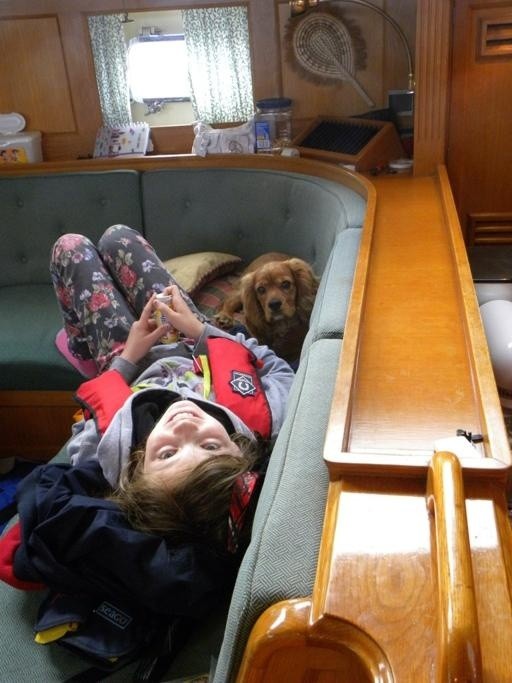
92;121;150;161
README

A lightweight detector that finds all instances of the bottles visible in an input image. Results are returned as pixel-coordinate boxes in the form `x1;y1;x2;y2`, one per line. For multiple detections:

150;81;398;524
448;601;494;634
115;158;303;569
155;293;179;344
253;98;292;154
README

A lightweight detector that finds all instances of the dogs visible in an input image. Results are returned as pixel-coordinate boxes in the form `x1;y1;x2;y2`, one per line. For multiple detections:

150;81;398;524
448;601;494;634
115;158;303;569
210;250;324;358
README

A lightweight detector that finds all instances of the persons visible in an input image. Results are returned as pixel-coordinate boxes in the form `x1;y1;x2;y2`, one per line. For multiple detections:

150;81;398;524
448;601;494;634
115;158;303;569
50;223;295;546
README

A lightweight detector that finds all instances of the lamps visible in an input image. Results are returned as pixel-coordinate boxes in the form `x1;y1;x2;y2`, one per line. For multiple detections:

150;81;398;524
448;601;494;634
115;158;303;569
291;0;415;92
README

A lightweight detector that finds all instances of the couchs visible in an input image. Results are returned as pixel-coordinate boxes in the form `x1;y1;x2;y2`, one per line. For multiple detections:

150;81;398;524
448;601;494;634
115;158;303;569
0;155;377;683
